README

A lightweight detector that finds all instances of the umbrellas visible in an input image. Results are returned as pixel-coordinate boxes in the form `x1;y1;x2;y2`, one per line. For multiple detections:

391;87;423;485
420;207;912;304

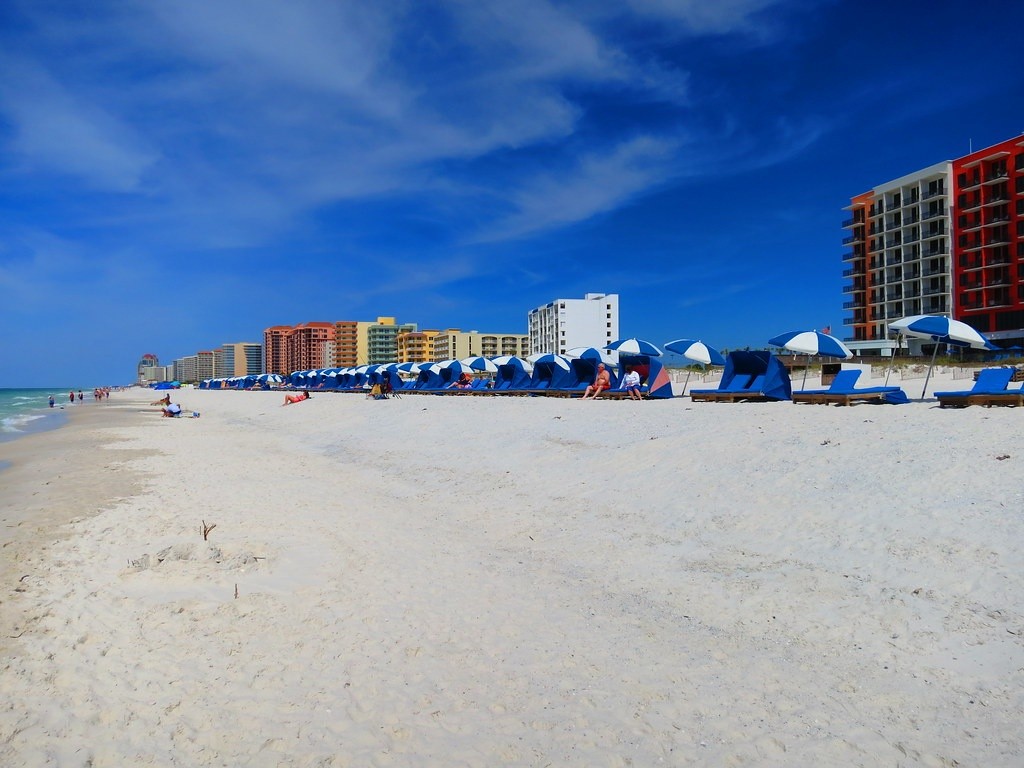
992;344;1004;350
768;328;853;391
1007;346;1023;349
664;338;725;396
604;337;664;356
888;314;992;398
197;347;618;386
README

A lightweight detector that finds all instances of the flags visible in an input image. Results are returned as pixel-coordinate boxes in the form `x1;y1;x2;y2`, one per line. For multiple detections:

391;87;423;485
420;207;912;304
822;325;830;334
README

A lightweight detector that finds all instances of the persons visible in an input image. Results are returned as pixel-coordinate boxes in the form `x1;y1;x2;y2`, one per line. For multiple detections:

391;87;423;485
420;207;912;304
78;390;83;403
448;373;474;389
617;365;643;401
282;390;309;406
160;393;170;404
69;392;74;403
313;378;327;388
578;363;611;400
166;401;182;417
280;379;286;388
251;381;260;390
48;394;54;407
94;386;112;402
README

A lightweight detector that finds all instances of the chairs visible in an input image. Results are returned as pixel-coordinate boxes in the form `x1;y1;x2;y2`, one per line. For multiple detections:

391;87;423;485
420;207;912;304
934;369;1023;409
206;381;649;399
690;374;766;403
793;370;901;407
